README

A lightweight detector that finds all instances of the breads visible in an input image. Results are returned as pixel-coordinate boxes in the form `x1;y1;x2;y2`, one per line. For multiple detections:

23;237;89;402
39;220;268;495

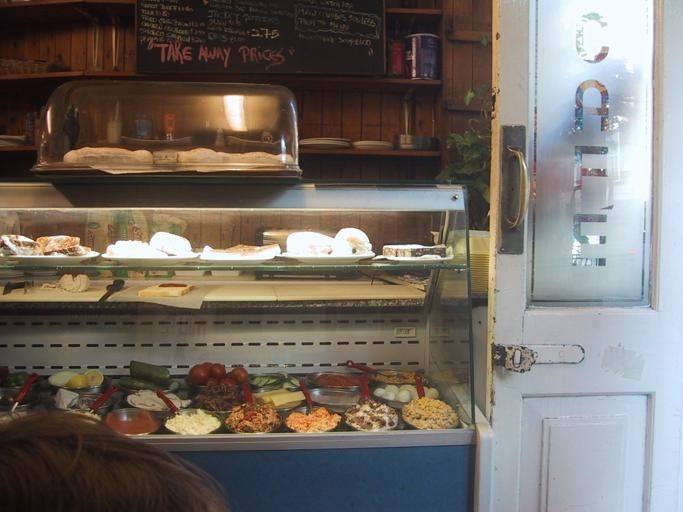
139;281;193;297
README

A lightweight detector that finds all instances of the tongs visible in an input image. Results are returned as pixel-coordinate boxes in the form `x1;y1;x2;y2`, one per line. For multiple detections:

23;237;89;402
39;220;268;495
3;280;34;296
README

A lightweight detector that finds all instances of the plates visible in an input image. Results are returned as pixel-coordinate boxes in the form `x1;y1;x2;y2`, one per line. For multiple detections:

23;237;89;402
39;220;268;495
1;251;101;266
199;254;277;266
375;254;454;268
298;137;395;151
101;253;198;267
274;254;375;267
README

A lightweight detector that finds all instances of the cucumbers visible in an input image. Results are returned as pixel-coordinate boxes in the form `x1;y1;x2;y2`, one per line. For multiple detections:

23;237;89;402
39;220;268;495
119;361;180;391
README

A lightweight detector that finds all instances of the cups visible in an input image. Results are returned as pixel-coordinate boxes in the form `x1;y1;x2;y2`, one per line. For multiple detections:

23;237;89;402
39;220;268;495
105;110;124;146
0;54;50;75
161;108;177;140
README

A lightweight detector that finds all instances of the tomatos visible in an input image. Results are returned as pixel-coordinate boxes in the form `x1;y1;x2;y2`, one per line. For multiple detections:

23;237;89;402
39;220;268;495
188;362;248;385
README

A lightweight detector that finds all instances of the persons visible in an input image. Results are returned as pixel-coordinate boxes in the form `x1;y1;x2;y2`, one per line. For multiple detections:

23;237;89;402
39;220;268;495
0;407;230;512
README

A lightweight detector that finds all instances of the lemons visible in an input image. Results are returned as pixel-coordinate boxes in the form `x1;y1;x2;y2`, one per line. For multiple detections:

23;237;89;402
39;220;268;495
65;374;88;386
84;370;104;387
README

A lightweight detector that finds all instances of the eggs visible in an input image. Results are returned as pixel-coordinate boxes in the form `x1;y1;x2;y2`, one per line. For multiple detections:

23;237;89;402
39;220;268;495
375;384;439;402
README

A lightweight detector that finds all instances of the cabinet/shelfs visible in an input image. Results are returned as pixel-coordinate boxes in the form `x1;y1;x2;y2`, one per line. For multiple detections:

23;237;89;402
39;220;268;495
2;183;475;511
1;0;442;180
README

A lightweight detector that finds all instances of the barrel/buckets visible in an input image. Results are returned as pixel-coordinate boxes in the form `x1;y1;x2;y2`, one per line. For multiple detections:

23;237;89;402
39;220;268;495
407;33;440;79
